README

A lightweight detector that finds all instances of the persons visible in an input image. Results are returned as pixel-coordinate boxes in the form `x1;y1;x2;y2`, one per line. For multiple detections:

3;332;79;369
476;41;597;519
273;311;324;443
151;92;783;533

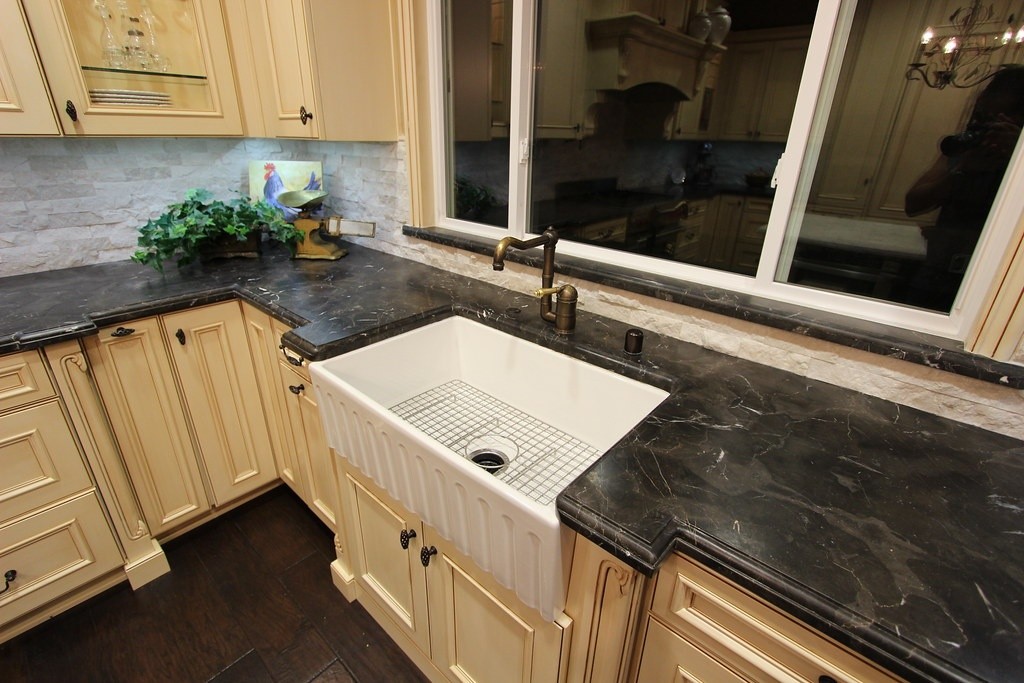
903;66;1024;304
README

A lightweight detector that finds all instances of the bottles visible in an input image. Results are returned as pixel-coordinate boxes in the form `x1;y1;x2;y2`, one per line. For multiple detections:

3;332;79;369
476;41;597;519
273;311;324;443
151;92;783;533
708;4;732;45
689;9;711;41
95;0;172;73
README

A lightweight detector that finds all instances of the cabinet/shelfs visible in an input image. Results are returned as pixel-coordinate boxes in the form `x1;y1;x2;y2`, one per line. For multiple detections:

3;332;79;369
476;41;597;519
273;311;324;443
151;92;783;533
0;0;408;143
489;0;1024;274
1;296;910;683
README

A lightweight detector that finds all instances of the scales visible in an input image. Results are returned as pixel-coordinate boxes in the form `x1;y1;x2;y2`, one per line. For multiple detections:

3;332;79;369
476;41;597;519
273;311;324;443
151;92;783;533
277;190;376;260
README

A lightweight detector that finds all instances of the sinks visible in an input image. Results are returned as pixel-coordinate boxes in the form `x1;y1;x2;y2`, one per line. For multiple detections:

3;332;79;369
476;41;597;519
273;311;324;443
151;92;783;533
309;304;680;623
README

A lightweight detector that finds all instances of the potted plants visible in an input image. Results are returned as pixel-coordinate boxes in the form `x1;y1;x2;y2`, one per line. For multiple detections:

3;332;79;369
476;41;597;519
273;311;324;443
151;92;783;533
131;188;306;275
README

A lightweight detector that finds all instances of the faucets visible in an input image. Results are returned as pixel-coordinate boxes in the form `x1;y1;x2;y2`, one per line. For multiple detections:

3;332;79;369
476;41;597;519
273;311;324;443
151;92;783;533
493;226;578;334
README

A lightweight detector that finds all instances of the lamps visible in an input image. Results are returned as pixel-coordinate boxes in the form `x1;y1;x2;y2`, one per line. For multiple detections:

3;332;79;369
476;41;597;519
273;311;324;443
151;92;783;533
900;0;1024;90
